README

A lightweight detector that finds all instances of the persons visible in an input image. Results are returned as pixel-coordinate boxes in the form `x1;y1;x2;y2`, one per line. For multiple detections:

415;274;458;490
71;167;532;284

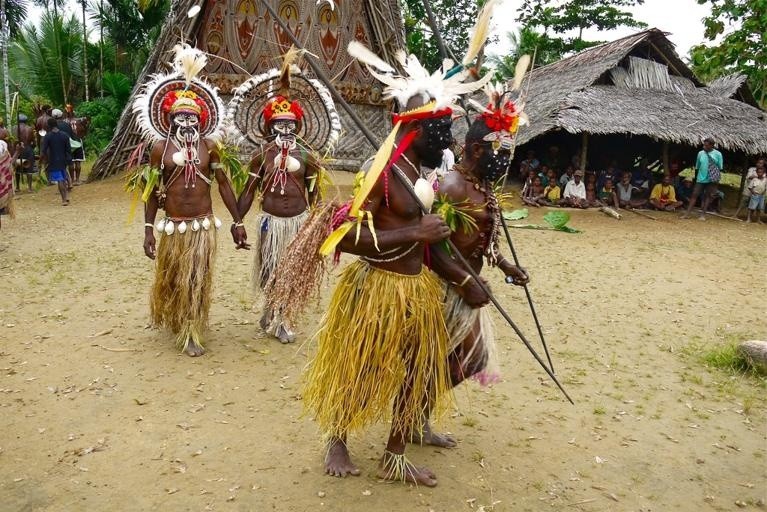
0;103;86;217
230;109;322;342
406;107;529;448
517;137;766;224
142;98;245;358
320;91;456;487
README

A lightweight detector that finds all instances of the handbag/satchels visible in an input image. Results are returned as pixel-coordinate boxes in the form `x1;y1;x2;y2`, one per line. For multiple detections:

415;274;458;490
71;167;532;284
709;157;721;183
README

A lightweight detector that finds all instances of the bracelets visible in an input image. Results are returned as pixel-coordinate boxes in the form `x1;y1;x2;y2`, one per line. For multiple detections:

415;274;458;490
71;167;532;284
144;221;153;228
495;257;505;268
458;273;472;288
236;221;244;228
232;222;239;225
